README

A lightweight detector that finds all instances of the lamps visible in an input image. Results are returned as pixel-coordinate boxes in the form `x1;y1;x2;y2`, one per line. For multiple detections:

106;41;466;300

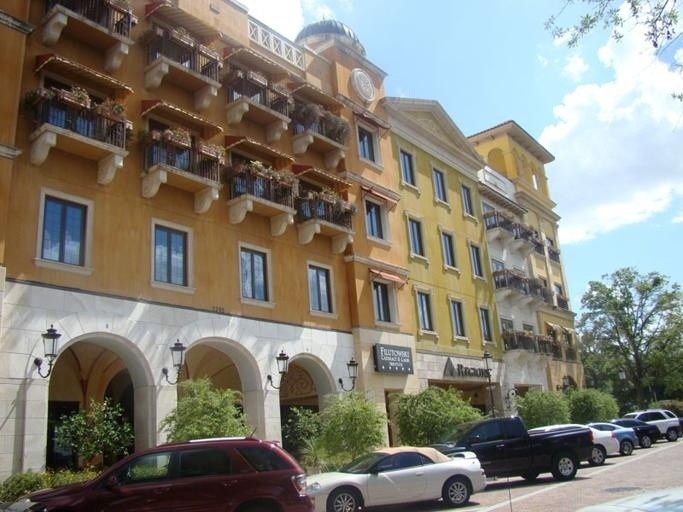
268;350;290;390
162;338;187;385
339;357;358;391
34;324;62;378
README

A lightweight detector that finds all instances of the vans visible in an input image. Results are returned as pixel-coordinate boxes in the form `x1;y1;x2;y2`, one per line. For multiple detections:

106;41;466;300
3;436;316;512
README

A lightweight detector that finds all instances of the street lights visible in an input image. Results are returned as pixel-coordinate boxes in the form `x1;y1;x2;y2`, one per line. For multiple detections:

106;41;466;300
481;348;496;420
618;369;626;408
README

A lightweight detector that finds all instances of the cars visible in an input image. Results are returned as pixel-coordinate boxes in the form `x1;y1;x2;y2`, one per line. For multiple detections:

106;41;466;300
306;446;488;511
622;408;683;441
611;419;661;448
586;423;641;456
528;423;621;466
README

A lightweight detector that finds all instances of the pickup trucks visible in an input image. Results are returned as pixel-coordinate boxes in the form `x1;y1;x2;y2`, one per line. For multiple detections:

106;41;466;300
439;416;594;483
